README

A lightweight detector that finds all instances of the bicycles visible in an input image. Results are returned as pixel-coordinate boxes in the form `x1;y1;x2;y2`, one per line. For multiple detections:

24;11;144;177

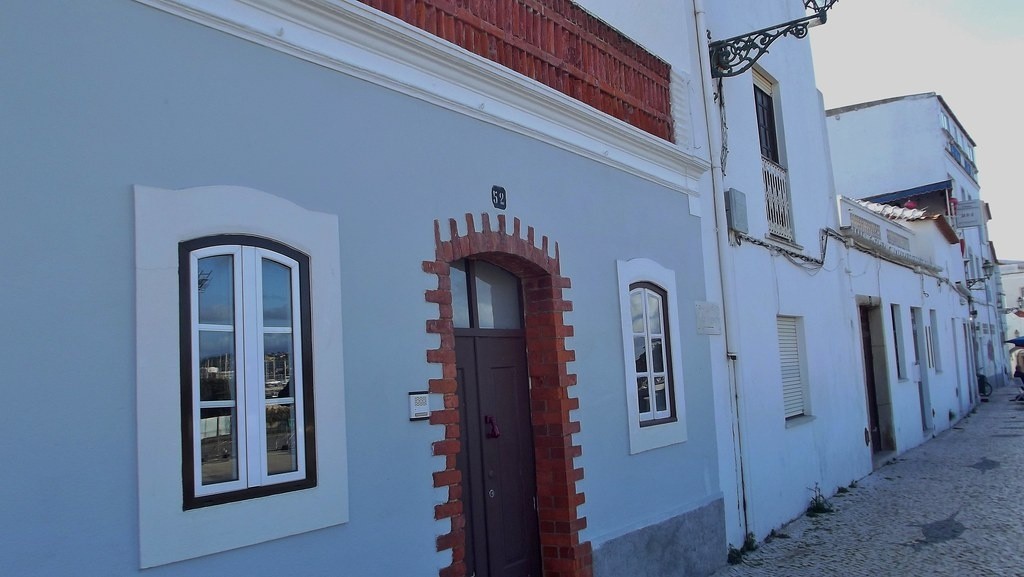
977;367;993;398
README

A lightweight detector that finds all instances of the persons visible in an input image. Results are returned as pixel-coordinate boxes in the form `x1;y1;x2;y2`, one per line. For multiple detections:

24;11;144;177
1014;365;1024;392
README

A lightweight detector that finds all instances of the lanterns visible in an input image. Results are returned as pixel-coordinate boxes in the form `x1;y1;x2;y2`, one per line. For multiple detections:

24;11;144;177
904;198;917;209
